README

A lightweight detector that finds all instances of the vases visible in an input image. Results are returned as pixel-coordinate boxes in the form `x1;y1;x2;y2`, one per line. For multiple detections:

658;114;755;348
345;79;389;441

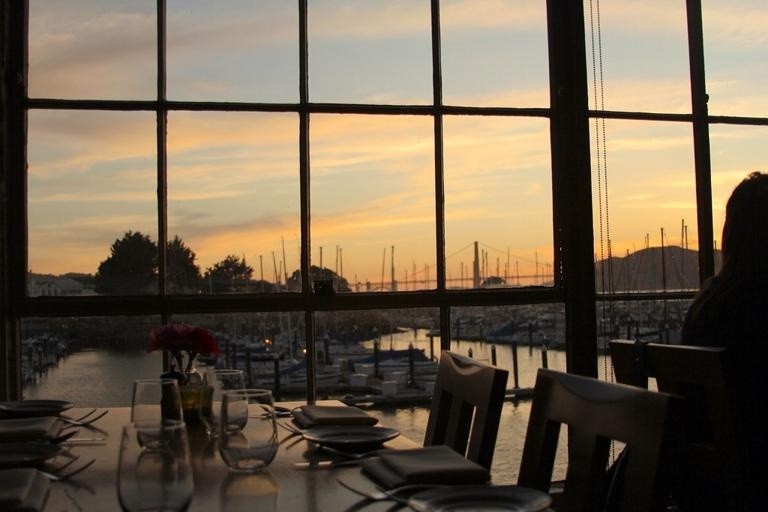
160;384;215;468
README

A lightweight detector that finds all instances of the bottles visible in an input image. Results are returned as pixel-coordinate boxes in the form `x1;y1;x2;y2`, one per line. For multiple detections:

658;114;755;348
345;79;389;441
114;418;195;512
128;377;186;455
197;368;280;472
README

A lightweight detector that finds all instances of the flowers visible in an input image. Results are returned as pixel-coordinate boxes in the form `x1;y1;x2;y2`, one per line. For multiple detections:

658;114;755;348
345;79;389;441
144;322;223;409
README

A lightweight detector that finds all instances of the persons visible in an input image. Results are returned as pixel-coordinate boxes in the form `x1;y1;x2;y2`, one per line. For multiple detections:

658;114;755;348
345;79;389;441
671;164;766;509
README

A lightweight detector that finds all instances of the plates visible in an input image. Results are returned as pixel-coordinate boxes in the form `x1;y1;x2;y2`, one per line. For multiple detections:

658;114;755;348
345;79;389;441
0;442;61;467
0;399;75;416
407;484;553;511
300;424;401;452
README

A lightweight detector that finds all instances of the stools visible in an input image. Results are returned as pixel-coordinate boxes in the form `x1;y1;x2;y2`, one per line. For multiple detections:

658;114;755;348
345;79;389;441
424;347;513;481
513;365;689;508
607;340;728;381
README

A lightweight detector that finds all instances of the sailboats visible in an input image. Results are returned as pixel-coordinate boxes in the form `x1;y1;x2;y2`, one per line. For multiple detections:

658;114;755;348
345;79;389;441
21;269;69;386
187;219;697;402
